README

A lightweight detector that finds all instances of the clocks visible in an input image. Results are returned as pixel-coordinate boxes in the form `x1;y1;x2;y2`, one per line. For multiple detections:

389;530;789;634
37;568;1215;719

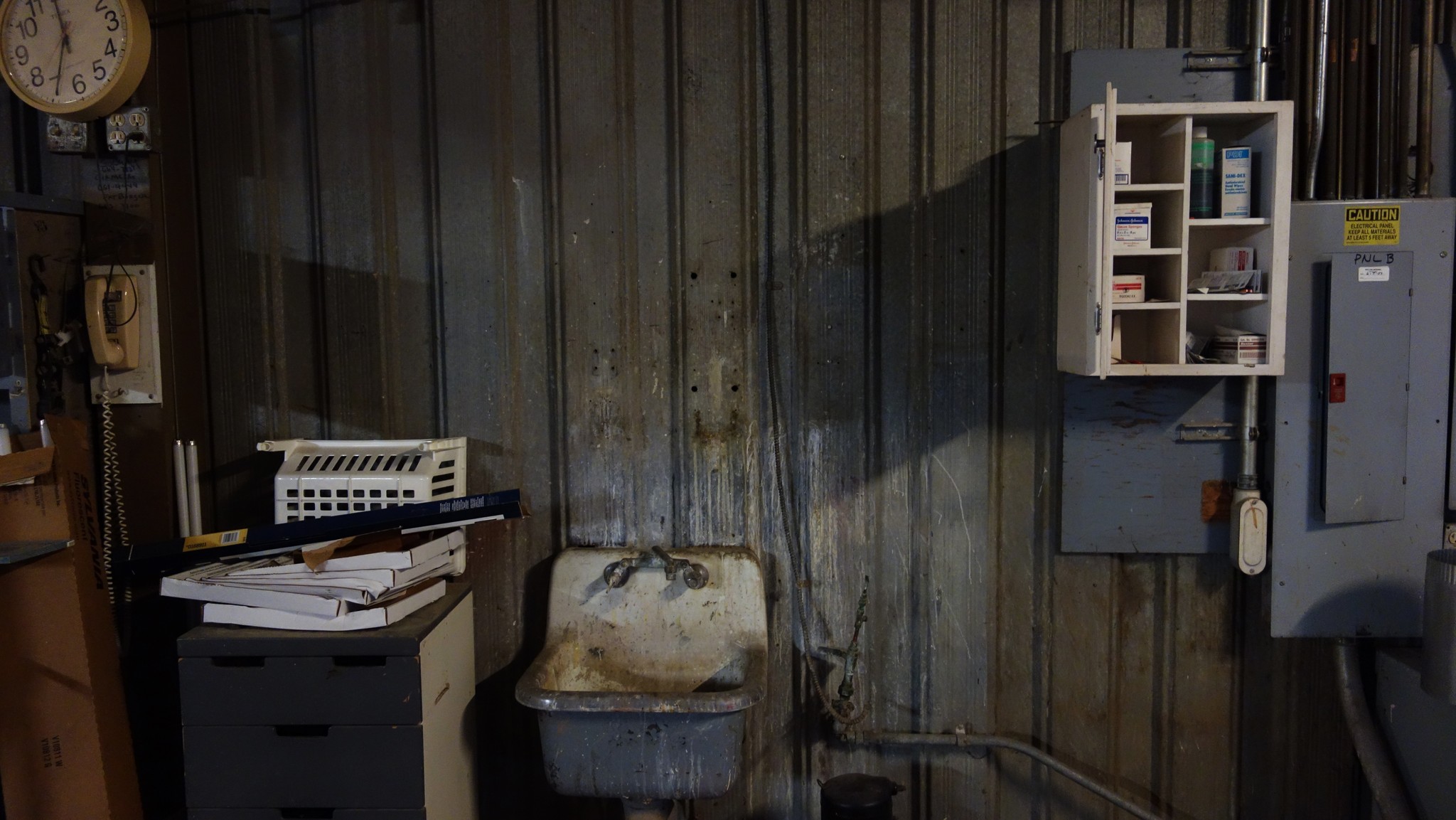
0;0;152;123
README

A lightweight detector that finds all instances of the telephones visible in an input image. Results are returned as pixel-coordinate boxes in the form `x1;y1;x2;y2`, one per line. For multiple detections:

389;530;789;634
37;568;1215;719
83;273;140;372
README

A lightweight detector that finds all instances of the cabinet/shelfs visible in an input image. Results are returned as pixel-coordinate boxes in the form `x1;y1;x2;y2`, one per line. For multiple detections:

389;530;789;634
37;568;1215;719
177;580;479;820
1056;99;1295;378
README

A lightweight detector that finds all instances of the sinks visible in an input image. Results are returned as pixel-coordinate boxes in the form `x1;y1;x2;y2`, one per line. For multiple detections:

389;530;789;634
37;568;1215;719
515;542;770;801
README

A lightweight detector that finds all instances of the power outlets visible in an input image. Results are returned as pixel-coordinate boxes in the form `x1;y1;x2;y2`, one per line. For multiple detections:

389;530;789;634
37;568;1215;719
107;106;153;153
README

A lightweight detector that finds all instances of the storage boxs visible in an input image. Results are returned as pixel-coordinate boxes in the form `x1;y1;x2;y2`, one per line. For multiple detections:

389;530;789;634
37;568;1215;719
160;489;533;631
0;414;144;820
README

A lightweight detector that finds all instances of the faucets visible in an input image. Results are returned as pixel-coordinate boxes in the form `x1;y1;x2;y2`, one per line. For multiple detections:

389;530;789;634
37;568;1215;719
605;544;709;591
